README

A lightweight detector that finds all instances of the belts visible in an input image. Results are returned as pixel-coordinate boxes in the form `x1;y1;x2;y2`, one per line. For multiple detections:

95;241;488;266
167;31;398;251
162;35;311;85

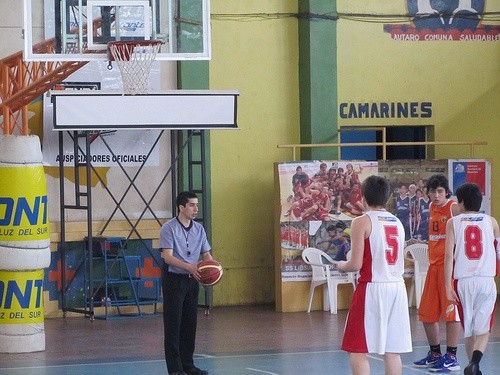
166;272;193;278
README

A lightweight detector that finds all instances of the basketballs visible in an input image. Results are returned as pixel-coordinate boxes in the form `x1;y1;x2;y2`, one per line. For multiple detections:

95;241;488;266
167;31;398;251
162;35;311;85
195;259;224;287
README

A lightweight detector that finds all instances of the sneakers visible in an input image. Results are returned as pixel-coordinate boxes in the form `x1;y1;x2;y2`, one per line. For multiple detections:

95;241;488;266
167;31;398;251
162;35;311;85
284;210;291;216
343;202;353;209
428;352;460;372
413;351;443;367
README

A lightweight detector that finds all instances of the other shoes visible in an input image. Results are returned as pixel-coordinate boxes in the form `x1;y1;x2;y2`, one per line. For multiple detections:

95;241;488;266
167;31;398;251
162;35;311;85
336;208;341;213
297;217;302;220
464;361;482;375
170;371;188;375
185;367;207;375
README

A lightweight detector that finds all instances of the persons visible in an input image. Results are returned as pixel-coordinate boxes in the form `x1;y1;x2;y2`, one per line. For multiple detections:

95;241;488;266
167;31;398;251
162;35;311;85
159;191;222;375
395;178;432;256
413;175;463;373
284;163;367;221
336;175;413;375
444;182;500;375
281;224;308;248
317;220;352;261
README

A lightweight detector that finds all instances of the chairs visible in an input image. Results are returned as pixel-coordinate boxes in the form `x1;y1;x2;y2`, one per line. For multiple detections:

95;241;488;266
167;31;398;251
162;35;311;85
302;248;361;315
404;243;461;322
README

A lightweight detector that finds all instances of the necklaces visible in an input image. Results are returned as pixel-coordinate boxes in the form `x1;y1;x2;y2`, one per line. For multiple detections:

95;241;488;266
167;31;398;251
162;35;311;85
177;216;192;257
